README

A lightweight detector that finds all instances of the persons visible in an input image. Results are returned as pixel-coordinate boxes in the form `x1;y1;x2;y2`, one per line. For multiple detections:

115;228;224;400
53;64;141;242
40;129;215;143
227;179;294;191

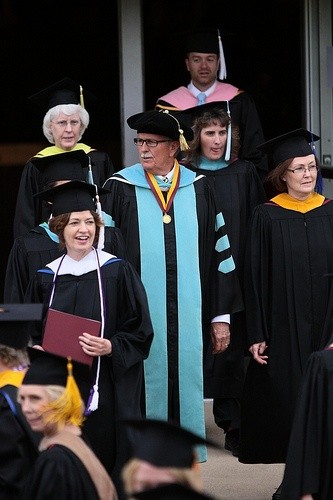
4;150;134;303
283;339;333;500
0;180;224;500
99;108;243;471
13;77;115;239
152;19;269;168
175;100;268;280
244;128;333;464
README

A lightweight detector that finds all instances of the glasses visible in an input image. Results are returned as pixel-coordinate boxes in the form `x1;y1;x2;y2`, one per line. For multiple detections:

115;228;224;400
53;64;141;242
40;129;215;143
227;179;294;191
57;119;78;127
286;165;319;174
134;138;177;147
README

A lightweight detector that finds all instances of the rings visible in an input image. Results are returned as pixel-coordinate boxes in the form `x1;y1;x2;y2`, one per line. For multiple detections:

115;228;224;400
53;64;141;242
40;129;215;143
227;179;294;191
225;344;228;346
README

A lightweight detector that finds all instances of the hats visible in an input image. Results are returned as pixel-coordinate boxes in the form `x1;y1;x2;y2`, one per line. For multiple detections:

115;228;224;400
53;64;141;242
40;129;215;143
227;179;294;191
119;419;225;469
30;149;94;184
126;108;194;151
27;76;97;120
182;26;227;81
21;345;92;431
0;301;45;345
34;181;105;249
255;128;320;159
178;101;239;128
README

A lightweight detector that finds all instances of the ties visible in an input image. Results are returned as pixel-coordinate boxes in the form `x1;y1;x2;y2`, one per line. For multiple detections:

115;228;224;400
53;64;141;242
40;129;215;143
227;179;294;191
196;93;207;105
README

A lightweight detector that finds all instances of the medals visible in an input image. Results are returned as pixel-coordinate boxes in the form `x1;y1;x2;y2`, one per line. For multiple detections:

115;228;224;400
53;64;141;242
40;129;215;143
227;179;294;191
162;212;171;224
